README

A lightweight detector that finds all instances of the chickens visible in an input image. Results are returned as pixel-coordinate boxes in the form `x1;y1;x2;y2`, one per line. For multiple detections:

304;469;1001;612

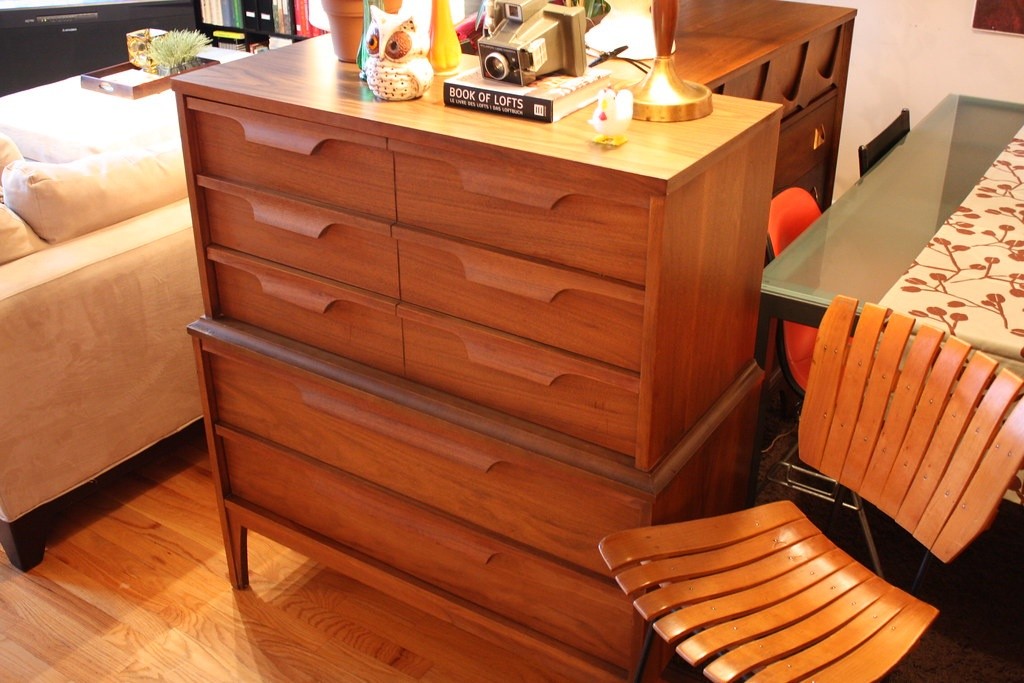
590;85;635;148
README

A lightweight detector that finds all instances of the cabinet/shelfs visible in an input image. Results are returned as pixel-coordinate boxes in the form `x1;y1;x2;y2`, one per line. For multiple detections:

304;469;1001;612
583;0;858;385
195;0;308;52
170;33;783;683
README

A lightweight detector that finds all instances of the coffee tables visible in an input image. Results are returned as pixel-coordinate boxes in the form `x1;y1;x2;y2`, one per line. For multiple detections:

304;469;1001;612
0;46;256;166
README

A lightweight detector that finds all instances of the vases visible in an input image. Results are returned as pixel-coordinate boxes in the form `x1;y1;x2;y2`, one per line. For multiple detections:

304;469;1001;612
321;0;404;62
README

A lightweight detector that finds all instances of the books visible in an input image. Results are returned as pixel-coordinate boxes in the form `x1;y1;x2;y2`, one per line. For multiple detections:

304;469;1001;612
200;0;329;55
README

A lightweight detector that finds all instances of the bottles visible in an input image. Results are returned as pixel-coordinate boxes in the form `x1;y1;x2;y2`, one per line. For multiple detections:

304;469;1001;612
427;0;462;74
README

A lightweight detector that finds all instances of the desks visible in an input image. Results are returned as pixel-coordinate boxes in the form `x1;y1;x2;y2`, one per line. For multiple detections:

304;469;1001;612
759;94;1024;587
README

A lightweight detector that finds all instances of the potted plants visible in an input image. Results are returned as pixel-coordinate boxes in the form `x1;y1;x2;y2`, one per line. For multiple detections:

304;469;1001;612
147;27;214;75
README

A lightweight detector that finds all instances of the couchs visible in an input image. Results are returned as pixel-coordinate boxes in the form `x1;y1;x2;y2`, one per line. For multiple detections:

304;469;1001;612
0;134;211;572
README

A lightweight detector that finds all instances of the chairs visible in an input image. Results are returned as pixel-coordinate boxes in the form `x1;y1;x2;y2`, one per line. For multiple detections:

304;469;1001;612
600;294;1024;683
748;191;885;583
